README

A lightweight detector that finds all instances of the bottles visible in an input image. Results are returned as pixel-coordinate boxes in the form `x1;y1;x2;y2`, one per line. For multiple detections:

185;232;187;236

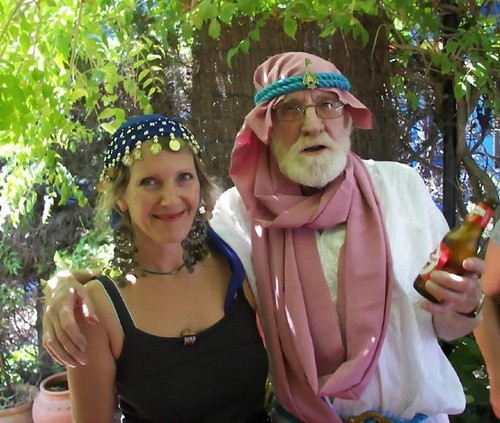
32;371;73;423
413;202;494;304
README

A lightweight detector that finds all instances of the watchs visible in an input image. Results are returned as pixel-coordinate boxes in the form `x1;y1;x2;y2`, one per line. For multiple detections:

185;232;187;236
454;287;486;318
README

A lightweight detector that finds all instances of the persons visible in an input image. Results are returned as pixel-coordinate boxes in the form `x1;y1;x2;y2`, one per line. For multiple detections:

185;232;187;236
475;221;500;416
44;48;488;423
69;114;272;423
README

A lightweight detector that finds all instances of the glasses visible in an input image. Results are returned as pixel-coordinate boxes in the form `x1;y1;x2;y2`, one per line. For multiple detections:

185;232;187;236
273;94;348;122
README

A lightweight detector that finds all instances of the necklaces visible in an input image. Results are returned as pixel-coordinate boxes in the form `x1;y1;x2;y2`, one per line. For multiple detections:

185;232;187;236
141;265;185;275
